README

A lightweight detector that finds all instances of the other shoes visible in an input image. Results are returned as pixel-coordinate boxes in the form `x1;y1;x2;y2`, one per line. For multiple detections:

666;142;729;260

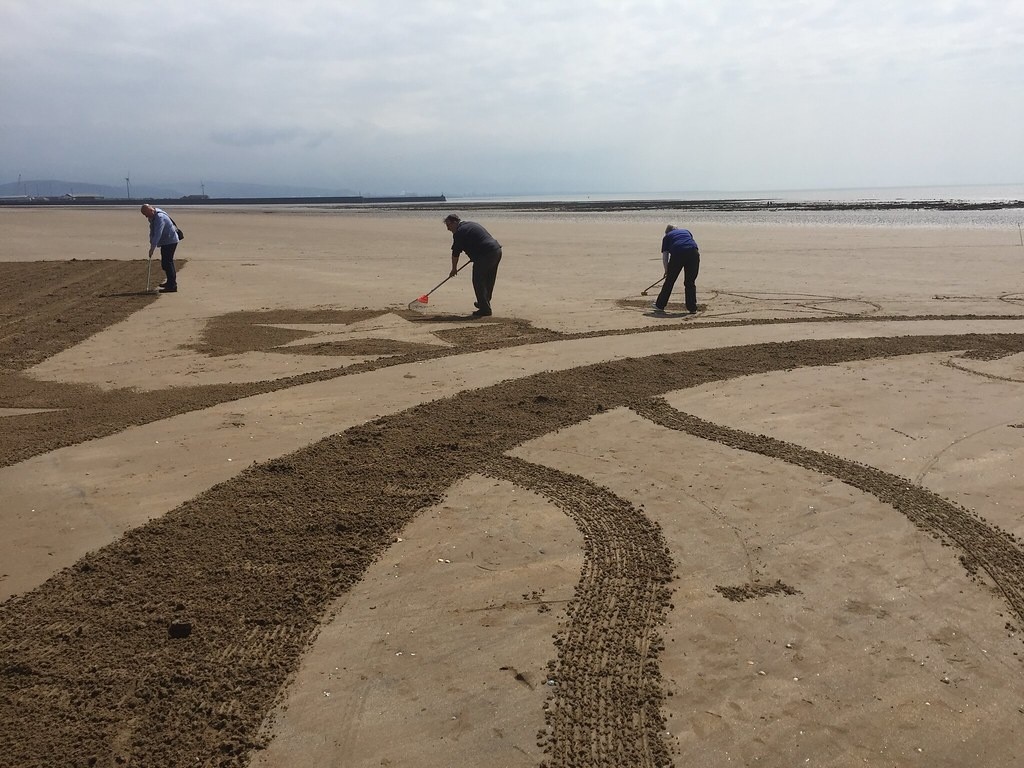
158;282;167;288
652;303;665;311
691;310;697;313
474;301;479;308
473;309;493;316
159;285;177;293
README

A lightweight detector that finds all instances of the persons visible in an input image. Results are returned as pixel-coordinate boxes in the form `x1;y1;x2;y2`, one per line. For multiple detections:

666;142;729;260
444;214;502;315
140;204;179;292
653;224;700;314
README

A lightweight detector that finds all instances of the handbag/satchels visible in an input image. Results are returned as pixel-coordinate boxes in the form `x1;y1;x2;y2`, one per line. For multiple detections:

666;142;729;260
175;229;184;240
